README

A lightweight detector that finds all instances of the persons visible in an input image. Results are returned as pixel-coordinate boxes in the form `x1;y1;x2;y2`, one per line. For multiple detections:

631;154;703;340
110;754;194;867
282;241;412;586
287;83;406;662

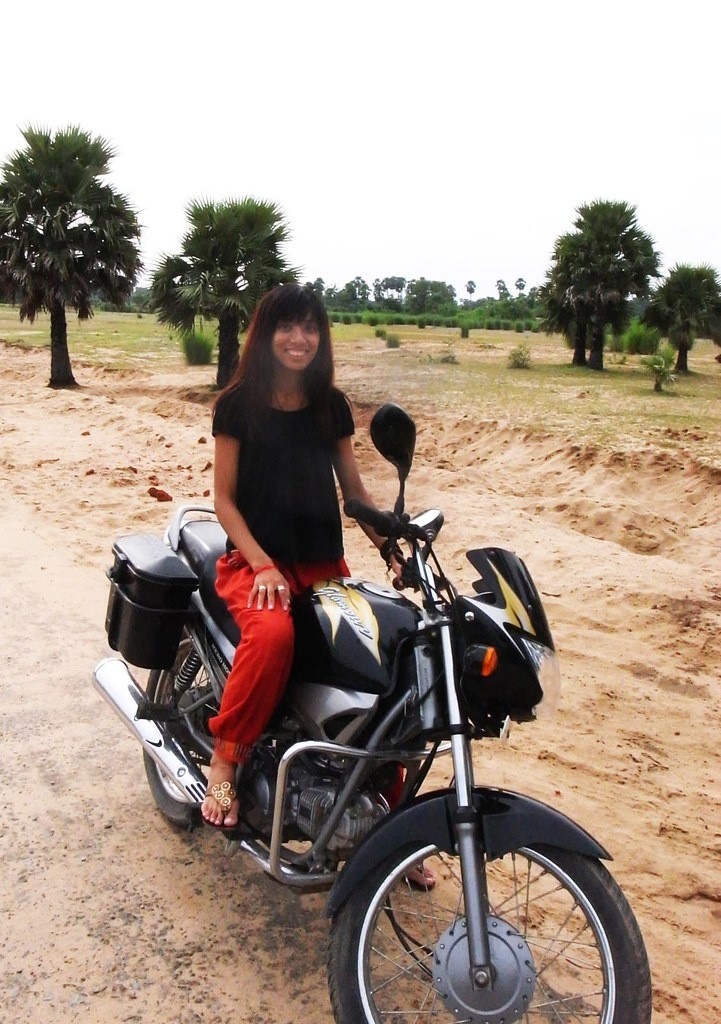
203;282;434;889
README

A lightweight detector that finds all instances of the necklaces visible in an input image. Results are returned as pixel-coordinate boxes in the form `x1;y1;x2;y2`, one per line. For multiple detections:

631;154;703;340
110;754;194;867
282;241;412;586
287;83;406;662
273;391;306;411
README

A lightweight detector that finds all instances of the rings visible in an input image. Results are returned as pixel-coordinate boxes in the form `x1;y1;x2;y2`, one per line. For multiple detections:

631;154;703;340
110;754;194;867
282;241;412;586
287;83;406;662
259;586;266;589
278;586;285;590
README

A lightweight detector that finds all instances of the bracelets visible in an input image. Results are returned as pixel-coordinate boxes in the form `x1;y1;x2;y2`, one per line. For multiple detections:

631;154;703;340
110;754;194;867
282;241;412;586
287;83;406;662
380;539;403;570
254;565;275;576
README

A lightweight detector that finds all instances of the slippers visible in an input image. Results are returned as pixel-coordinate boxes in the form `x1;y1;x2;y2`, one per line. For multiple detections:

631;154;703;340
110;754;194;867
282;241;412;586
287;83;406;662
201;772;240;831
403;866;435;892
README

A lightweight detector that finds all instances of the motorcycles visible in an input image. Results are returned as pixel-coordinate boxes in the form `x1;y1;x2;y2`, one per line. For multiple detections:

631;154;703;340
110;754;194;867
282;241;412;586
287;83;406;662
91;401;653;1024
